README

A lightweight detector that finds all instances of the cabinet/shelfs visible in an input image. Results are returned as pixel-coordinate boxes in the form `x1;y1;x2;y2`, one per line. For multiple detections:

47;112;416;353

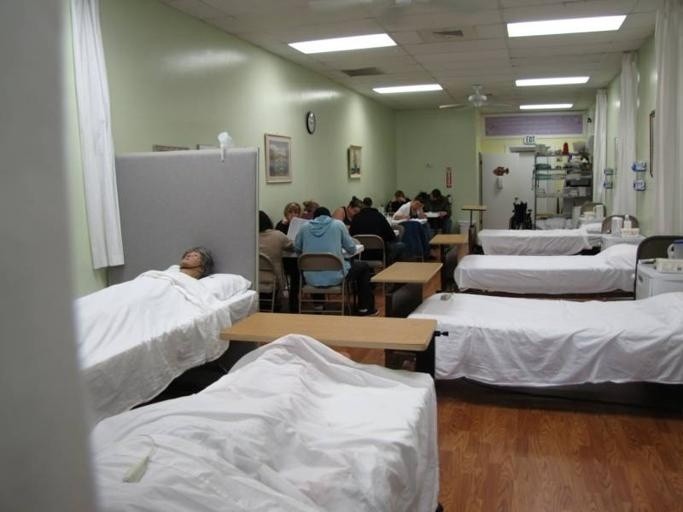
534;153;593;229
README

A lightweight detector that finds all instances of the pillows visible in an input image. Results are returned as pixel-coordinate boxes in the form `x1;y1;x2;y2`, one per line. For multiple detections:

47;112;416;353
164;264;250;298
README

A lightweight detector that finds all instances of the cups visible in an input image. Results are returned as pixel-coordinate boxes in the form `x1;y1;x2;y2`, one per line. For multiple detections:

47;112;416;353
582;205;605;221
655;239;682;273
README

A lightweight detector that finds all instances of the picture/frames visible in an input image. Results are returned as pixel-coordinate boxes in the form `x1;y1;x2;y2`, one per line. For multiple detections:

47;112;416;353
264;133;293;184
348;144;364;179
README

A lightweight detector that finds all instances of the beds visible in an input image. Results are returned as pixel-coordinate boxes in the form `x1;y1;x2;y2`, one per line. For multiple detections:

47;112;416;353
86;337;440;511
74;271;257;421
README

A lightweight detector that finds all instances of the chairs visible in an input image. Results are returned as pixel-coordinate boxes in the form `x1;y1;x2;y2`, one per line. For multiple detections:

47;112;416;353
352;234;385;296
297;252;350;316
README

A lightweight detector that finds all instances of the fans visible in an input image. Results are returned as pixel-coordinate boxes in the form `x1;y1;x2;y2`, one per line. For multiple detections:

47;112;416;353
439;85;513;109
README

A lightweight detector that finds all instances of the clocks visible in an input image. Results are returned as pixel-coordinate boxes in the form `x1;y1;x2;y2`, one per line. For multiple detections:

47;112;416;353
306;111;317;134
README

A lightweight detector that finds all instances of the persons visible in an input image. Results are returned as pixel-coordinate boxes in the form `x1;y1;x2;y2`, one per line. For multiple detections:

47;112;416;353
178;245;215;280
257;188;451;316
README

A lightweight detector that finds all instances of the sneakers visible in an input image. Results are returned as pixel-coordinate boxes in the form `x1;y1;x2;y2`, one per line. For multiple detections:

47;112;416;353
358;308;380;317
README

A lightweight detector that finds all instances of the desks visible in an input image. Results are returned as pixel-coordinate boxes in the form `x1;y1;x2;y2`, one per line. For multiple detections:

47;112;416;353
260;253;279;311
219;313;437;369
370;261;443;369
282;242;364;262
388;218;427;236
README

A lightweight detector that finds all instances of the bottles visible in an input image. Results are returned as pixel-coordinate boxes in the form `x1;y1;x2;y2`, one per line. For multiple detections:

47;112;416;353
562;142;569;154
610;213;640;236
